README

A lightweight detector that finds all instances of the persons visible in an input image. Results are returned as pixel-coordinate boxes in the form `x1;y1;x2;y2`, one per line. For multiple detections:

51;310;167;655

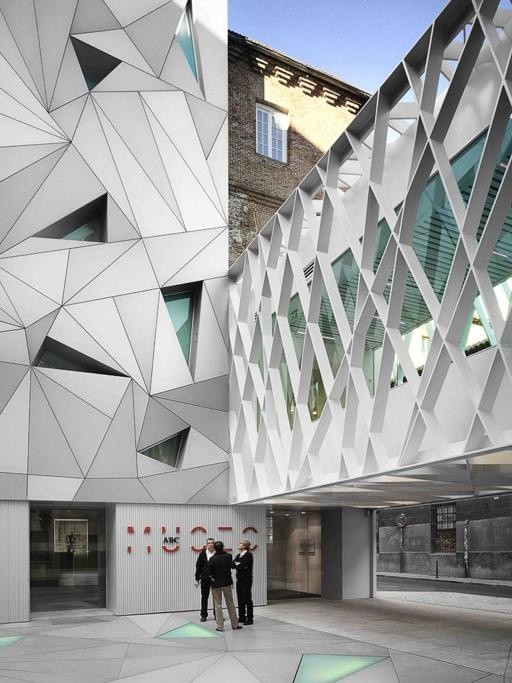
193;536;225;623
204;540;243;631
231;539;254;625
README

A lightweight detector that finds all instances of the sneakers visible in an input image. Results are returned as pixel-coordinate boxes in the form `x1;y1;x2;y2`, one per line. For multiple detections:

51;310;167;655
199;613;254;631
